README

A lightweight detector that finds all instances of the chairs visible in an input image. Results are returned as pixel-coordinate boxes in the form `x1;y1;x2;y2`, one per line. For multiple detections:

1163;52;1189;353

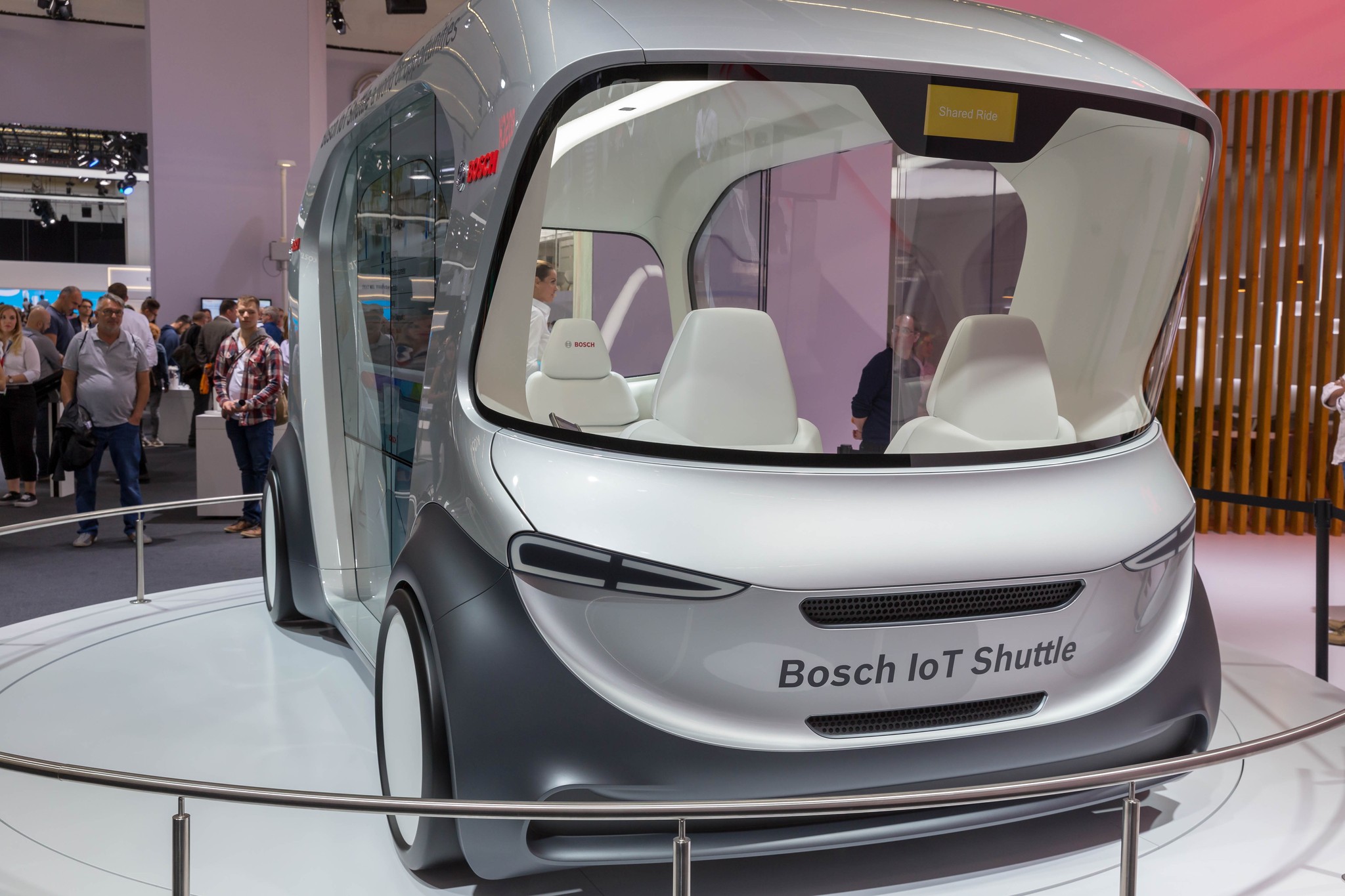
525;305;1074;454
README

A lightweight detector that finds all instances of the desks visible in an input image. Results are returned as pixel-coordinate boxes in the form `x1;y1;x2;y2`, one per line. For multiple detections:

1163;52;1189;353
195;413;286;516
157;386;194;444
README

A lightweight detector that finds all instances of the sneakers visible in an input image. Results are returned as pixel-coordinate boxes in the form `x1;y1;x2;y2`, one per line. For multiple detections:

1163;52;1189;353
14;493;38;507
128;532;152;544
0;491;21;505
73;533;99;546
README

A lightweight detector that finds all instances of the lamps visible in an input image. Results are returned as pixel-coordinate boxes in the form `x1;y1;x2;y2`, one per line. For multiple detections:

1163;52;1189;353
25;133;146;227
330;0;345;34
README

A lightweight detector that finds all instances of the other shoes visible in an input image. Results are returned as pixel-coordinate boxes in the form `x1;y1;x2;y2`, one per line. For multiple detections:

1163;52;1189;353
240;524;261;537
1328;619;1345;631
224;519;252;533
142;437;151;447
114;474;149;483
152;438;164;447
1329;631;1345;645
188;441;195;447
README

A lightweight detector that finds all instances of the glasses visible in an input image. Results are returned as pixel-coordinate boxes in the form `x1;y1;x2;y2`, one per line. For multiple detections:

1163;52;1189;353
149;309;157;316
892;327;916;337
261;313;267;317
96;308;123;317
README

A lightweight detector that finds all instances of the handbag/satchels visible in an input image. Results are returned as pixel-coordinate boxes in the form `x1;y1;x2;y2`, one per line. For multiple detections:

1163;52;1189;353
273;391;289;426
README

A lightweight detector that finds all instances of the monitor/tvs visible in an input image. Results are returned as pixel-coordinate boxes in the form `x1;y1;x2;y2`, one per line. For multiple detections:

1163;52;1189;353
201;297;272;328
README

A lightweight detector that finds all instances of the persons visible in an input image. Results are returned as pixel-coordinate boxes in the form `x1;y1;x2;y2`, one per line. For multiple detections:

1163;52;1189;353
359;302;456;494
851;313;922;454
527;260;559;378
1320;373;1345;646
0;283;293;546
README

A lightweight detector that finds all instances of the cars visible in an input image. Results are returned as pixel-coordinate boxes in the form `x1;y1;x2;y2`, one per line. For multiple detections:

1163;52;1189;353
259;0;1225;880
